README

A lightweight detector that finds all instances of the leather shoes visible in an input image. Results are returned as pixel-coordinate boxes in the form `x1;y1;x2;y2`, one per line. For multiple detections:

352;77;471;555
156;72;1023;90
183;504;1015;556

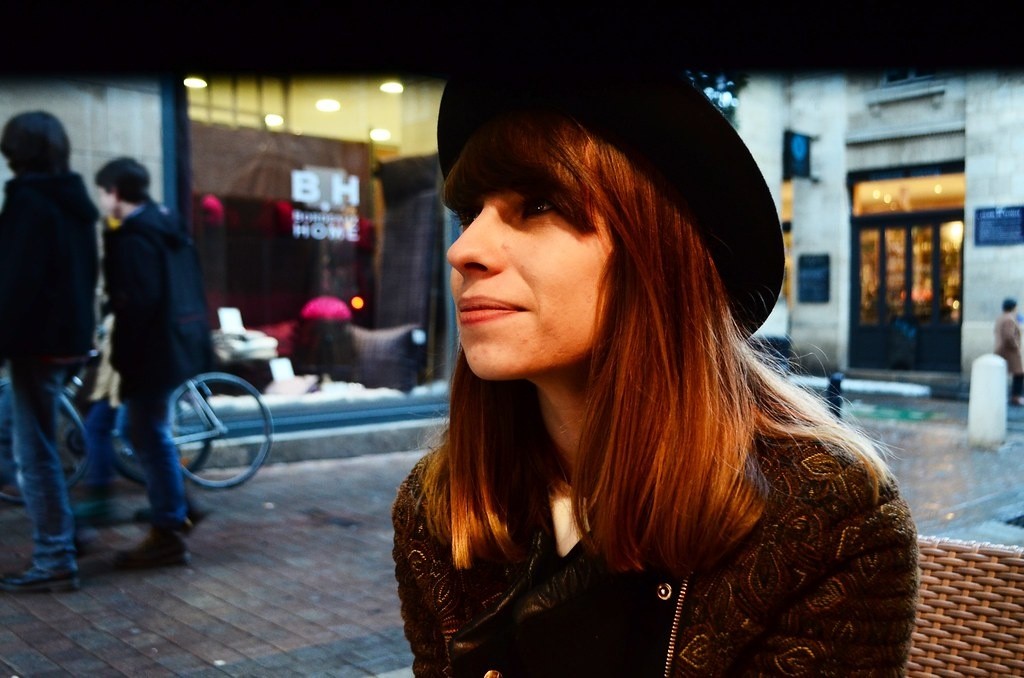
0;557;80;592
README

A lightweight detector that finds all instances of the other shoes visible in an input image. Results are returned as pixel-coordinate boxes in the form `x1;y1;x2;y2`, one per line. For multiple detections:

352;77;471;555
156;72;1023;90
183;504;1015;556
133;497;205;525
112;527;193;570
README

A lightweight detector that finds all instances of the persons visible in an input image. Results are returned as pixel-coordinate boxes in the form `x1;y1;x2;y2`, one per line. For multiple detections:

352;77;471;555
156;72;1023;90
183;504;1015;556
0;110;101;592
95;155;216;567
393;52;916;678
994;297;1024;407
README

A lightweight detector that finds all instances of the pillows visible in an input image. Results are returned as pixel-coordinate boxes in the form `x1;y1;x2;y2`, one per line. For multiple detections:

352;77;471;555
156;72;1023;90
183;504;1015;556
332;322;421;390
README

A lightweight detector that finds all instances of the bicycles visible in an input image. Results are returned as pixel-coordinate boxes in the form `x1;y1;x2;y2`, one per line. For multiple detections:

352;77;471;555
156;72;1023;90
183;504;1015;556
1;326;274;505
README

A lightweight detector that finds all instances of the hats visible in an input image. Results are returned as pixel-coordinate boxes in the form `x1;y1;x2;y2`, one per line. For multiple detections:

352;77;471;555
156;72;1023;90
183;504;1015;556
438;13;785;341
